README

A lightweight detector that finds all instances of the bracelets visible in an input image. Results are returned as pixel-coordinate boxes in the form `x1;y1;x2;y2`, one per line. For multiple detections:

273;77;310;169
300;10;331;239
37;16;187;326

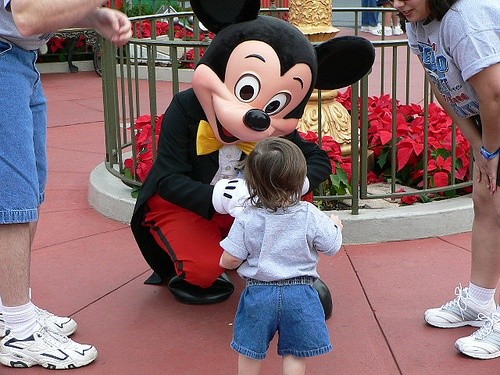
480;146;500;160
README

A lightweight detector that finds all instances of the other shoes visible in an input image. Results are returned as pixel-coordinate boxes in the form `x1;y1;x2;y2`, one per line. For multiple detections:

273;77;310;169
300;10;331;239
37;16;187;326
392;26;404;35
369;23;382;34
372;27;392;36
361;25;370;32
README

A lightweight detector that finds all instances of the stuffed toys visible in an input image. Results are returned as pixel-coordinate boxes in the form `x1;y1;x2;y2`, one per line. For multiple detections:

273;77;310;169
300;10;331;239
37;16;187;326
130;0;375;321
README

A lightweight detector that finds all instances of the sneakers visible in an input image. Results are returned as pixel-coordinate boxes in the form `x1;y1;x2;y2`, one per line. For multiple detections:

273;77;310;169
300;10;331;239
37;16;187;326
425;286;497;328
0;318;97;369
454;312;500;359
0;304;78;337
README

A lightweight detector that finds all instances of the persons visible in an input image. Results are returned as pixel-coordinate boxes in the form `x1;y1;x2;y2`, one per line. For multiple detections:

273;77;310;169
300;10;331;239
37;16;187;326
0;0;132;370
389;0;500;360
218;137;343;375
359;0;404;37
